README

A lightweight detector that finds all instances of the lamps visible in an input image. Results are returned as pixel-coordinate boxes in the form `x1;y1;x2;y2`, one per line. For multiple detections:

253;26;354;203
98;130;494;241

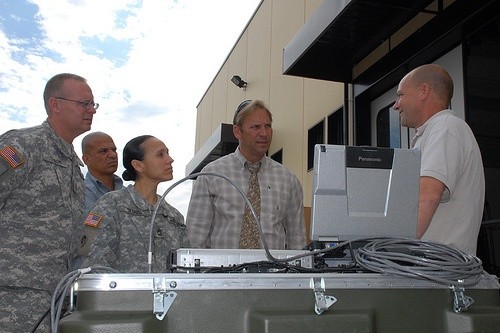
231;75;247;89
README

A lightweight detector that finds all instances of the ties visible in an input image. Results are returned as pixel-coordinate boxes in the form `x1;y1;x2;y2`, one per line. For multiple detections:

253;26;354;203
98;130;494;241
239;162;262;249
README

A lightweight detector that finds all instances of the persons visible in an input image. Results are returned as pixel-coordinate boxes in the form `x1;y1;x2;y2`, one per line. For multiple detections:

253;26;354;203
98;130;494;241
0;73;95;333
185;99;306;249
82;131;124;216
389;63;486;257
78;134;187;274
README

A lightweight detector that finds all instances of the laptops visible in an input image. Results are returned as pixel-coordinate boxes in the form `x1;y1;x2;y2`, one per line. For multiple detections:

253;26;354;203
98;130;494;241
299;144;422;250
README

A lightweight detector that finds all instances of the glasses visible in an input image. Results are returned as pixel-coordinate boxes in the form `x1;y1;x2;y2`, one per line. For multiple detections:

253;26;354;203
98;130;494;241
55;96;100;110
